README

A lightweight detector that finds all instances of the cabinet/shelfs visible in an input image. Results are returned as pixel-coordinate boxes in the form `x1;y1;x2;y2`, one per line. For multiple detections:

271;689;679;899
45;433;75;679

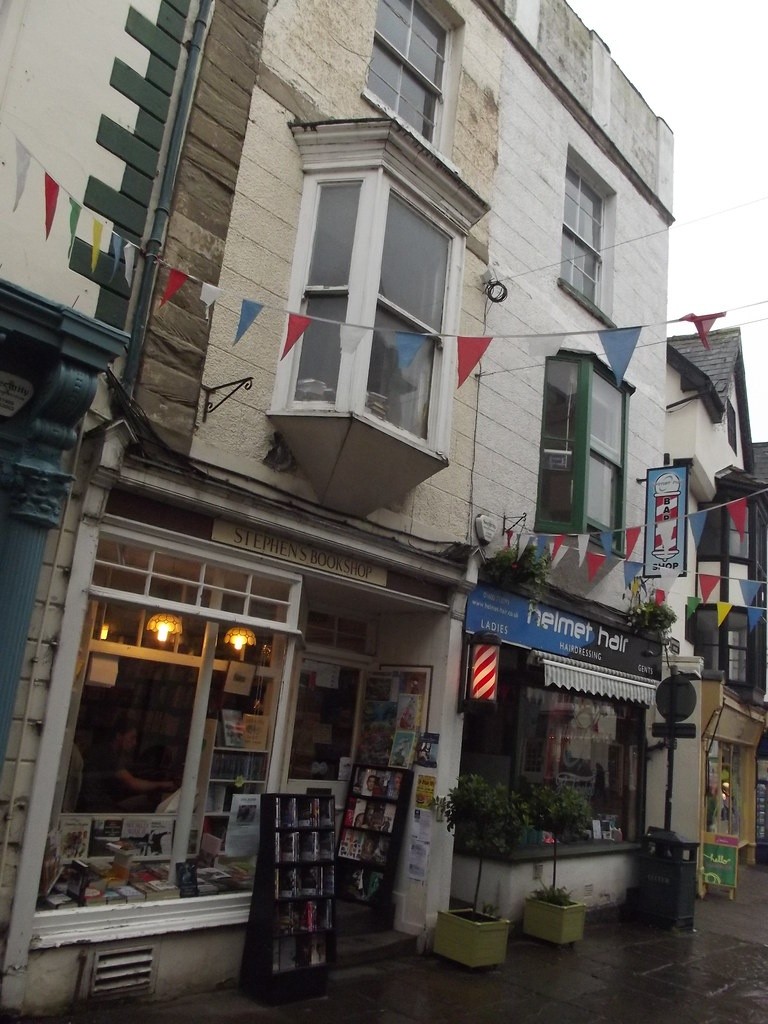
335;763;416;933
239;792;340;1010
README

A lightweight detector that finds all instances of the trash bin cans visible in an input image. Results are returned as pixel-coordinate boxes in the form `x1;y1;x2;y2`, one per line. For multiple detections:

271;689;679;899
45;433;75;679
641;830;702;931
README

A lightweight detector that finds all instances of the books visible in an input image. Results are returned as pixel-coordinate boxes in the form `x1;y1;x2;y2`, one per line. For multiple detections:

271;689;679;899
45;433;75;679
40;815;235;910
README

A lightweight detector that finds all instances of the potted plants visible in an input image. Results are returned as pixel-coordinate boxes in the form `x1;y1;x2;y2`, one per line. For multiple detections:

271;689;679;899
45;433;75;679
525;781;592;946
492;542;552;612
432;773;534;970
628;597;679;633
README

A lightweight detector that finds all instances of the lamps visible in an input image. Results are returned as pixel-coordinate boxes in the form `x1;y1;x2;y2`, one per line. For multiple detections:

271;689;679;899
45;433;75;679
144;612;183;643
224;625;257;651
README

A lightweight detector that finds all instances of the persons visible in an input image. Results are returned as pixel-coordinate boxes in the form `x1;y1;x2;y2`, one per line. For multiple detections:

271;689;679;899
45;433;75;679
382;817;391;833
92;716;177;813
354;813;364;828
366;775;376;791
291;707;328;768
